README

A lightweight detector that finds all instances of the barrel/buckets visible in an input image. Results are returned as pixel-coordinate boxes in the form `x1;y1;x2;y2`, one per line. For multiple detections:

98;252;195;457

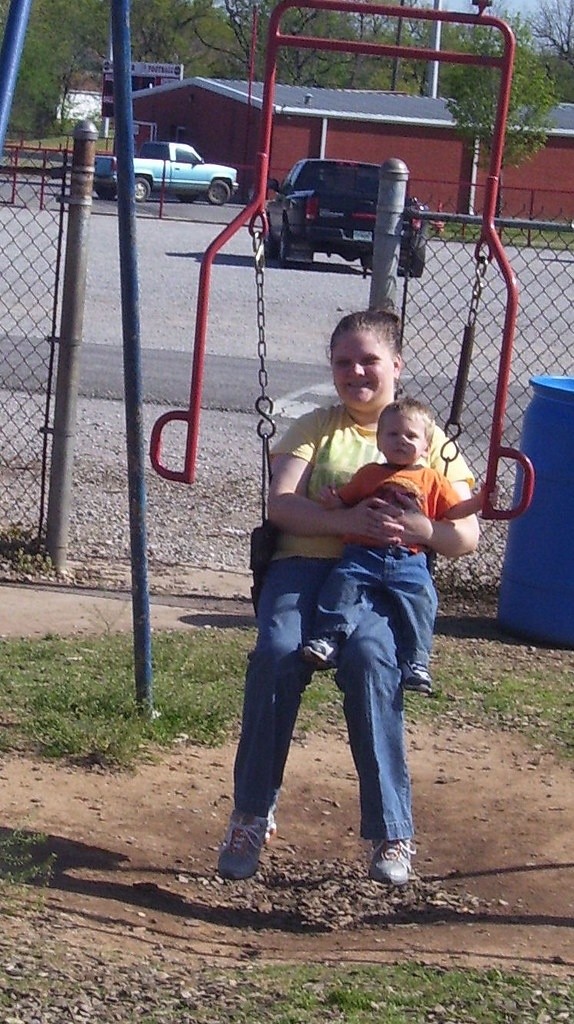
495;375;574;649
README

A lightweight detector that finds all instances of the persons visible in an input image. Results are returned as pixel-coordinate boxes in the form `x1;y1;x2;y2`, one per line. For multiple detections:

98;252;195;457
216;311;481;886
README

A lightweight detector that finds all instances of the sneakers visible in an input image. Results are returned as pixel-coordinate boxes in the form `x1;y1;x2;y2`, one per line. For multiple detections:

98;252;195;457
302;635;339;670
218;809;277;880
370;838;417;885
402;662;433;694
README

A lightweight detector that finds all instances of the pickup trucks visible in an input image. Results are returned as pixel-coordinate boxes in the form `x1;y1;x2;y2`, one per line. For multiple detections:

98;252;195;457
265;158;427;278
95;141;239;204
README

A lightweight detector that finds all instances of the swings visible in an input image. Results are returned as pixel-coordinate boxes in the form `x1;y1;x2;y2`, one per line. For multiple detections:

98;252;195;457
248;229;489;675
